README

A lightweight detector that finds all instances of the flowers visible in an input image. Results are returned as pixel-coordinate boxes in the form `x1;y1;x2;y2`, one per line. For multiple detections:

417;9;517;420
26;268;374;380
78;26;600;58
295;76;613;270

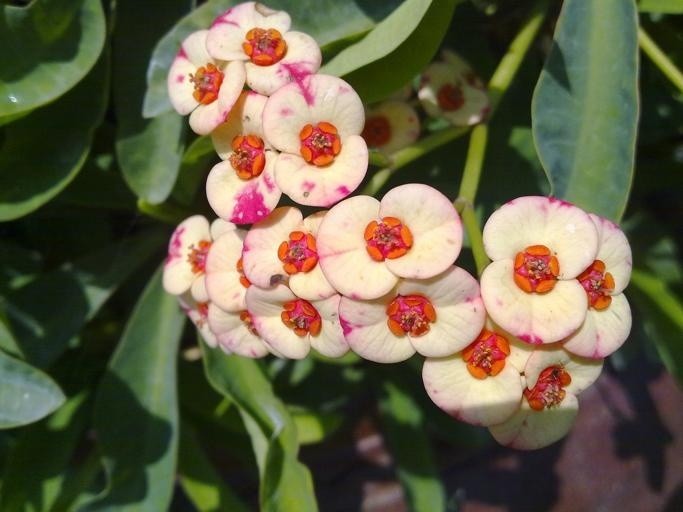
3;1;683;512
